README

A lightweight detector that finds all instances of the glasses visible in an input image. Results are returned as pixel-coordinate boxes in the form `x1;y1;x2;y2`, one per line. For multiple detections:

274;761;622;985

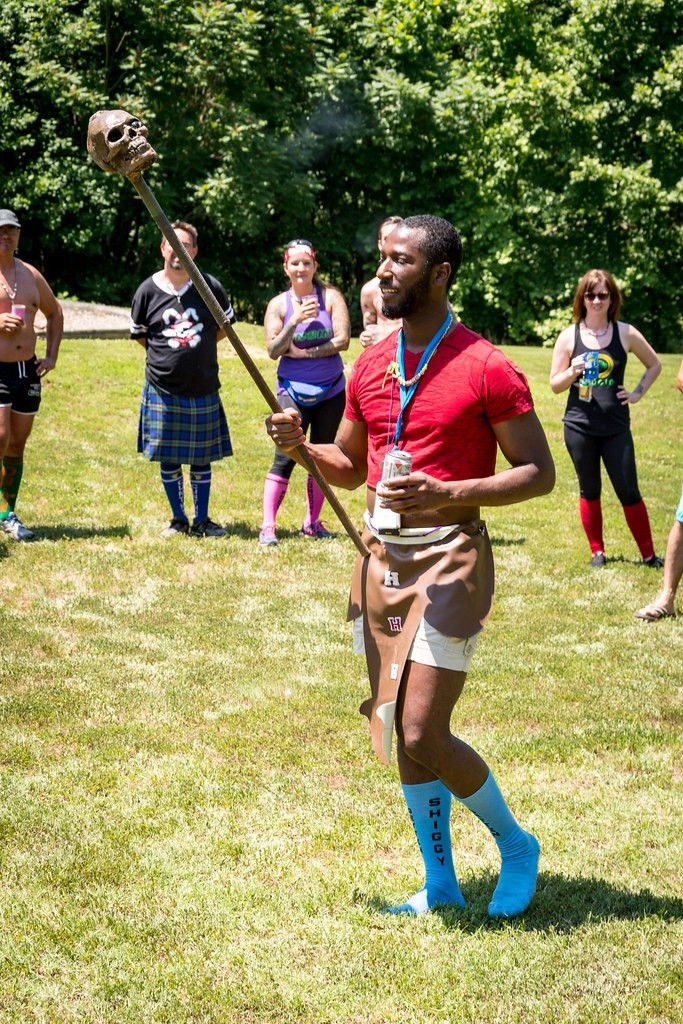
584;291;610;301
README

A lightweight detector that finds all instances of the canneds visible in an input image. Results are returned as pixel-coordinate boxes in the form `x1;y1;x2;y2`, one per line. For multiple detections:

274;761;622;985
381;449;412;489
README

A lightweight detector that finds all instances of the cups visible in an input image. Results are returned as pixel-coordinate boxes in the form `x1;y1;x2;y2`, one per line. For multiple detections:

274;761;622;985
12;304;26;327
301;294;318;318
366;324;382;346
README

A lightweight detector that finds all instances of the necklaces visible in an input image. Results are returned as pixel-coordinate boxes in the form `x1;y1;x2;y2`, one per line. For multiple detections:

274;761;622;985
381;312;453;390
583;316;610;337
288;283;316;304
1;256;17;302
163;276;183;304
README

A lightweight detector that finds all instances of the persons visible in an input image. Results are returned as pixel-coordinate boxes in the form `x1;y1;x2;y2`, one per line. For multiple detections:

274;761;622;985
361;217;402;350
0;210;63;544
264;213;557;929
259;238;353;549
550;269;665;570
632;354;682;621
130;219;236;541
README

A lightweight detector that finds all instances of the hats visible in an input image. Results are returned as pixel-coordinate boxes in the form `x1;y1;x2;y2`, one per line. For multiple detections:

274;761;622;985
0;209;21;227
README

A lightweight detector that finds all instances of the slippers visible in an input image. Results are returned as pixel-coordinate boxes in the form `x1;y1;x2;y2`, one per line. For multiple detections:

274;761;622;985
634;604;676;622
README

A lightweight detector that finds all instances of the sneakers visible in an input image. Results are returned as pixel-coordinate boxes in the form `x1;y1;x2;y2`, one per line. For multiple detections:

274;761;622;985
646;555;664;568
0;512;34;540
299;518;337;540
191;517;227;539
259;527;278;548
589;553;607;566
161;516;189;538
